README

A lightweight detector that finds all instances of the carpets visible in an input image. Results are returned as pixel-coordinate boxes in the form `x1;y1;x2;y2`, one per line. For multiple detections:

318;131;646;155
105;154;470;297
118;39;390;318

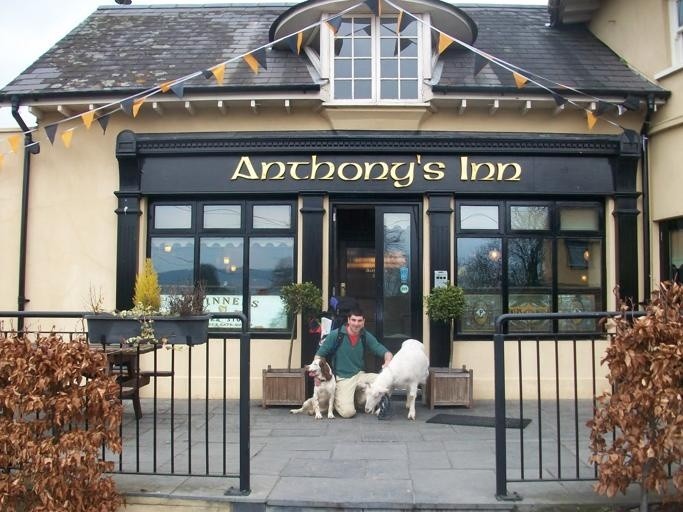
425;414;532;429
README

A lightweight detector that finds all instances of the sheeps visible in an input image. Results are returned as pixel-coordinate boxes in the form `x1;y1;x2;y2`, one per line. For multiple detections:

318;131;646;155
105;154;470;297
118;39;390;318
364;338;430;421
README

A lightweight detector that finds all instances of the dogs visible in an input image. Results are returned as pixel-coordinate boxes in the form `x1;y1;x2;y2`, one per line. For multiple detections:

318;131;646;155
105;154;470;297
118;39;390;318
288;357;336;421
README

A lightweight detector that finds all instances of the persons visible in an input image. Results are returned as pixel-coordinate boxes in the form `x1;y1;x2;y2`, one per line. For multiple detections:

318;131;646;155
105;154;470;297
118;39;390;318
310;308;393;417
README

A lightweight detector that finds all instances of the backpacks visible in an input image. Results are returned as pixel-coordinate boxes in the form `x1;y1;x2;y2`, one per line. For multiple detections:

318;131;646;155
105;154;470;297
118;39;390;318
308;296;366;356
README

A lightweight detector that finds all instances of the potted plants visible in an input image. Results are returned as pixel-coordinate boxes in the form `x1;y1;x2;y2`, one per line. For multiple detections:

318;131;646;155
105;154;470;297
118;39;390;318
261;281;323;410
85;259;209;349
424;284;474;409
